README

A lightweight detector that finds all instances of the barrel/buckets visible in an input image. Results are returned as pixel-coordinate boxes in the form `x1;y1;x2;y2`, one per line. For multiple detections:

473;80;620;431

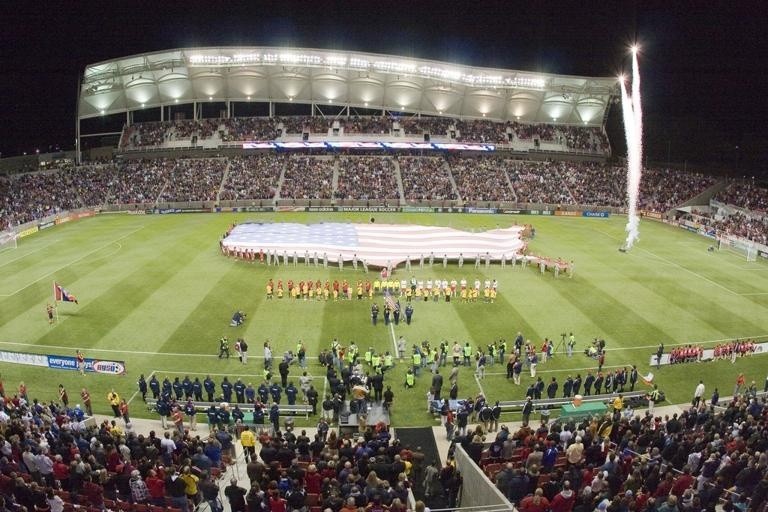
572;395;583;407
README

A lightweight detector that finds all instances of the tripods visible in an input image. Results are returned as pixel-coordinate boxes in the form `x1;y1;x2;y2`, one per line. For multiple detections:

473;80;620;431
554;337;568;355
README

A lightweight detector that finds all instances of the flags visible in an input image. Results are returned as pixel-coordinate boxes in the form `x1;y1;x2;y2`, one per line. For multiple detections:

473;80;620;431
54;282;78;305
383;291;397;311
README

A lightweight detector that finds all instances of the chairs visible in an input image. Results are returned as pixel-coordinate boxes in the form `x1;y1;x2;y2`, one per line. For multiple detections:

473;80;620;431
117;117;611;153
0;413;767;512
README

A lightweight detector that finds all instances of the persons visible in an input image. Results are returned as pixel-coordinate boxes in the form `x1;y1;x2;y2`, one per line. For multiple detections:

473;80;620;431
137;332;639;439
647;337;767;410
2;380;256;511
76;349;87;377
447;398;766;511
47;303;54;324
246;426;462;510
219;246;573;327
2;146;765;252
117;114;613;156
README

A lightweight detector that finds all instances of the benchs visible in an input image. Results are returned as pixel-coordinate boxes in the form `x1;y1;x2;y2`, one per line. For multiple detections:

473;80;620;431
144;396;314;423
494;389;647;413
